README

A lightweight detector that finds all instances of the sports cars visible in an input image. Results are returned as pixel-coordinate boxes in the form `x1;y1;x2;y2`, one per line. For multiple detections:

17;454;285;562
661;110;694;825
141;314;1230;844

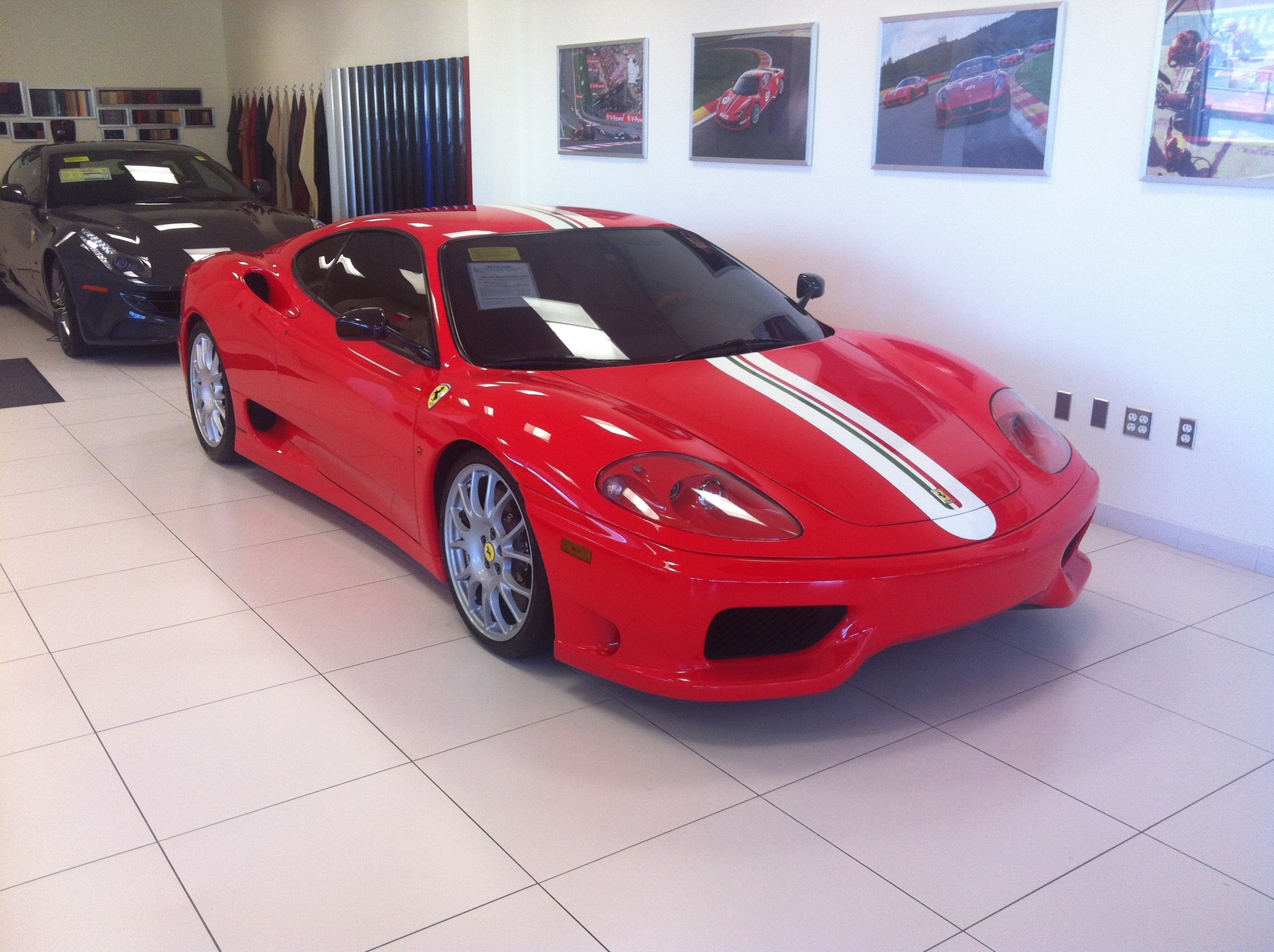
175;202;1102;701
934;53;1011;128
1029;37;1055;53
712;66;786;131
883;76;930;108
998;48;1026;67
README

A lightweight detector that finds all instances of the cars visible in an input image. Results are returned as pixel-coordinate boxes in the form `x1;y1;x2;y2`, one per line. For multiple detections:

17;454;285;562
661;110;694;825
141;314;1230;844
0;136;331;359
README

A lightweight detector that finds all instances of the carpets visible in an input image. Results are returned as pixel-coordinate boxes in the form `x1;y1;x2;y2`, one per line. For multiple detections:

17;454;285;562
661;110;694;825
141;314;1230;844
0;357;65;408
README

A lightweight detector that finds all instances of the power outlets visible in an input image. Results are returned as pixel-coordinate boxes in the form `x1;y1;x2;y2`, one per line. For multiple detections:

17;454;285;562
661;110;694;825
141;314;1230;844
1177;417;1197;449
1123;407;1154;440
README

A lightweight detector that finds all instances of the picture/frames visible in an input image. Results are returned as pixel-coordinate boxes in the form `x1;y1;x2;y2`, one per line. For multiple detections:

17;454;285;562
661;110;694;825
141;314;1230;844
129;108;182;128
867;0;1067;176
96;87;204;108
26;86;98;120
136;128;181;143
0;79;28;117
96;106;129;128
182;106;216;128
9;120;49;142
101;127;128;141
556;37;650;159
0;120;11;137
1139;0;1274;189
687;22;819;166
